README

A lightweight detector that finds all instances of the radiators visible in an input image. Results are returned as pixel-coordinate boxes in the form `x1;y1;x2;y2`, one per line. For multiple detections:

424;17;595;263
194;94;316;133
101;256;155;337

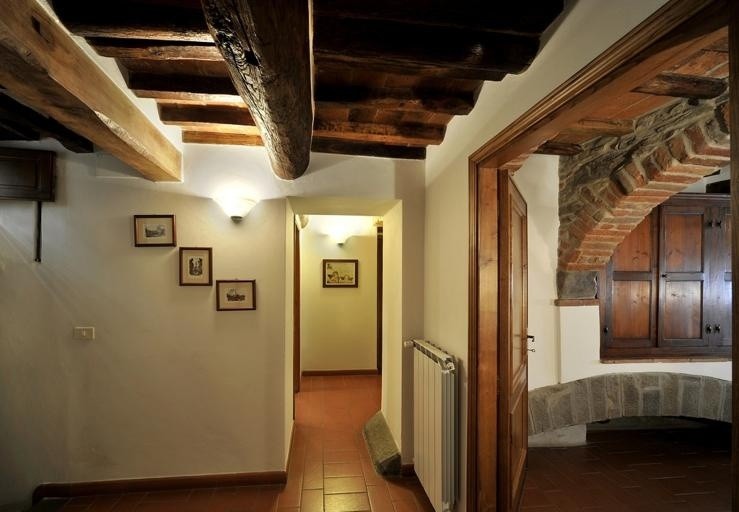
402;336;464;511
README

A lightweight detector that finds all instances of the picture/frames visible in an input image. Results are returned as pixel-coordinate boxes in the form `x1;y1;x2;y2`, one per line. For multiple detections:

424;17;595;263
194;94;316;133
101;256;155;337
320;257;361;290
132;212;257;312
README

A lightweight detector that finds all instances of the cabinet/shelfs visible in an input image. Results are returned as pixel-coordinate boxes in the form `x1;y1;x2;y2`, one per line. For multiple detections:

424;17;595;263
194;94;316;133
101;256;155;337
598;192;733;360
